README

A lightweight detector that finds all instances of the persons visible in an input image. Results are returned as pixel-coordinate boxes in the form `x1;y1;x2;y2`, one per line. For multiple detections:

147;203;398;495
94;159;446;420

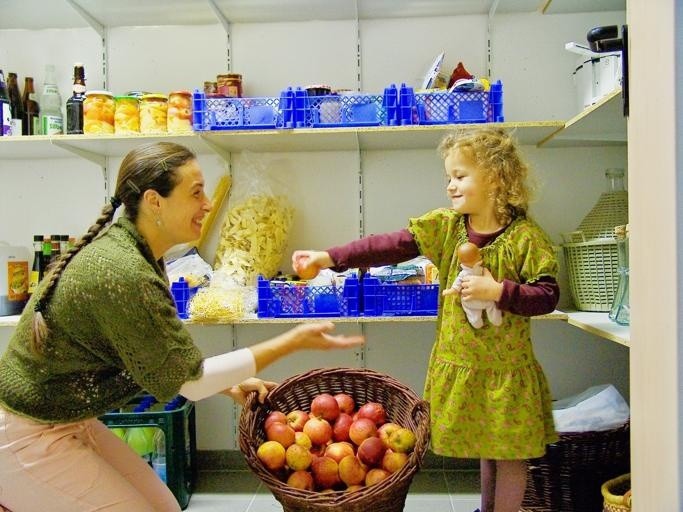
441;243;503;329
291;123;561;512
0;142;366;512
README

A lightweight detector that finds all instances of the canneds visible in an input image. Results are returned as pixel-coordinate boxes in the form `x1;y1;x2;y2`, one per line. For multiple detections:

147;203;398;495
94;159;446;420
82;91;193;135
303;84;352;127
204;72;243;98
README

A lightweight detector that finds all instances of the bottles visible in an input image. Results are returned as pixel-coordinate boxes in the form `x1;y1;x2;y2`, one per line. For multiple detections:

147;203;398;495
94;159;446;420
605;166;625;192
101;395;191;484
607;222;629;324
0;69;12;137
22;76;41;136
27;234;77;303
65;63;89;134
39;64;64;135
6;72;25;136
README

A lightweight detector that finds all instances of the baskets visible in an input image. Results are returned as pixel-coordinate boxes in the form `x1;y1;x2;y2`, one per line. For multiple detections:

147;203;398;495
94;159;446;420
399;78;502;126
190;78;502;133
92;392;200;511
510;398;631;512
560;230;624;313
295;84;399;128
165;271;440;319
190;86;296;131
239;365;431;512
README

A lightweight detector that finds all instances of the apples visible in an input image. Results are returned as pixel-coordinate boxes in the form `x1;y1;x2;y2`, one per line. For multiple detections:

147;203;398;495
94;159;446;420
296;256;320;280
256;393;416;498
